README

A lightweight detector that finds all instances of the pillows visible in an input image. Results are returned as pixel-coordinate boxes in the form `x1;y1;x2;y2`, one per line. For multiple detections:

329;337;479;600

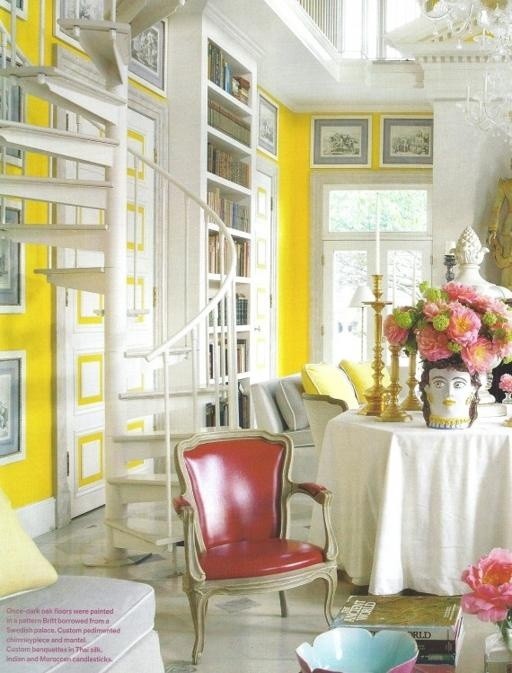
276;376;309;430
0;490;56;601
302;363;358;408
341;360;392;404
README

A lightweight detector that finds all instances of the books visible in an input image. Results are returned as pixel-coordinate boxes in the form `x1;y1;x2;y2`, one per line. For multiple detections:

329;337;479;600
209;134;249;184
209;87;249;145
208;287;247;326
209;41;251;104
206;176;248;233
332;595;465;672
209;338;246;377
209;228;247;277
205;391;249;429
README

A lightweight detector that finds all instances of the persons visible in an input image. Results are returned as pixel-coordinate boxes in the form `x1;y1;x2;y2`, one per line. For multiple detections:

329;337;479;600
486;159;511;288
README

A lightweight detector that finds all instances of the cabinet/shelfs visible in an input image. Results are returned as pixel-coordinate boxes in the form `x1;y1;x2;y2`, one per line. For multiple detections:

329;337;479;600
201;38;250;431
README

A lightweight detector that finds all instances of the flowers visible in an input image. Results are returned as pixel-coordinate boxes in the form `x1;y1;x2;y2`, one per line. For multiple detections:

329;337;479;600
498;374;512;394
459;547;512;651
384;278;512;374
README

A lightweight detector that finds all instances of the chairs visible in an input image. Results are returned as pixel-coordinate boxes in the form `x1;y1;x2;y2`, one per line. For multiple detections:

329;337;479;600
170;428;341;667
302;394;349;456
251;367;424;447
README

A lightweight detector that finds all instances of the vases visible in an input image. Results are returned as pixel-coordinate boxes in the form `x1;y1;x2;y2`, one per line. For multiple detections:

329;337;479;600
485;630;512;665
501;393;512;427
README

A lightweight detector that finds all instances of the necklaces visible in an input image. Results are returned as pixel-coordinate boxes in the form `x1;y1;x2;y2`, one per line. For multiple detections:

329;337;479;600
427;415;471;426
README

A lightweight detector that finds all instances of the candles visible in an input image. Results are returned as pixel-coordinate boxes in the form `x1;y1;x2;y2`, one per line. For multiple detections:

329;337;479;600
412;251;416;305
392;266;396;309
374;192;380;274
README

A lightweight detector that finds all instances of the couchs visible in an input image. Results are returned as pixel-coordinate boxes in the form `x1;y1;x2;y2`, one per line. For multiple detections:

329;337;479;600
1;481;167;673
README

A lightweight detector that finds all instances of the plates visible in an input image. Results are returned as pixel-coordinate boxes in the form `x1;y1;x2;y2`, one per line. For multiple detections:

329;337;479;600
295;627;419;673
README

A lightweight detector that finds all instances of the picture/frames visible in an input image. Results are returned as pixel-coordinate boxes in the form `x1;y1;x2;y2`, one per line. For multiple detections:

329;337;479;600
0;48;29;169
257;91;280;162
310;115;373;171
53;1;168;96
0;0;29;22
0;198;26;313
0;350;28;467
378;114;434;168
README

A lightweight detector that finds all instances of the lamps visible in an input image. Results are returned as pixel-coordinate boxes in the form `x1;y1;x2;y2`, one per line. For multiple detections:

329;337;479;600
349;286;373;362
420;0;512;146
